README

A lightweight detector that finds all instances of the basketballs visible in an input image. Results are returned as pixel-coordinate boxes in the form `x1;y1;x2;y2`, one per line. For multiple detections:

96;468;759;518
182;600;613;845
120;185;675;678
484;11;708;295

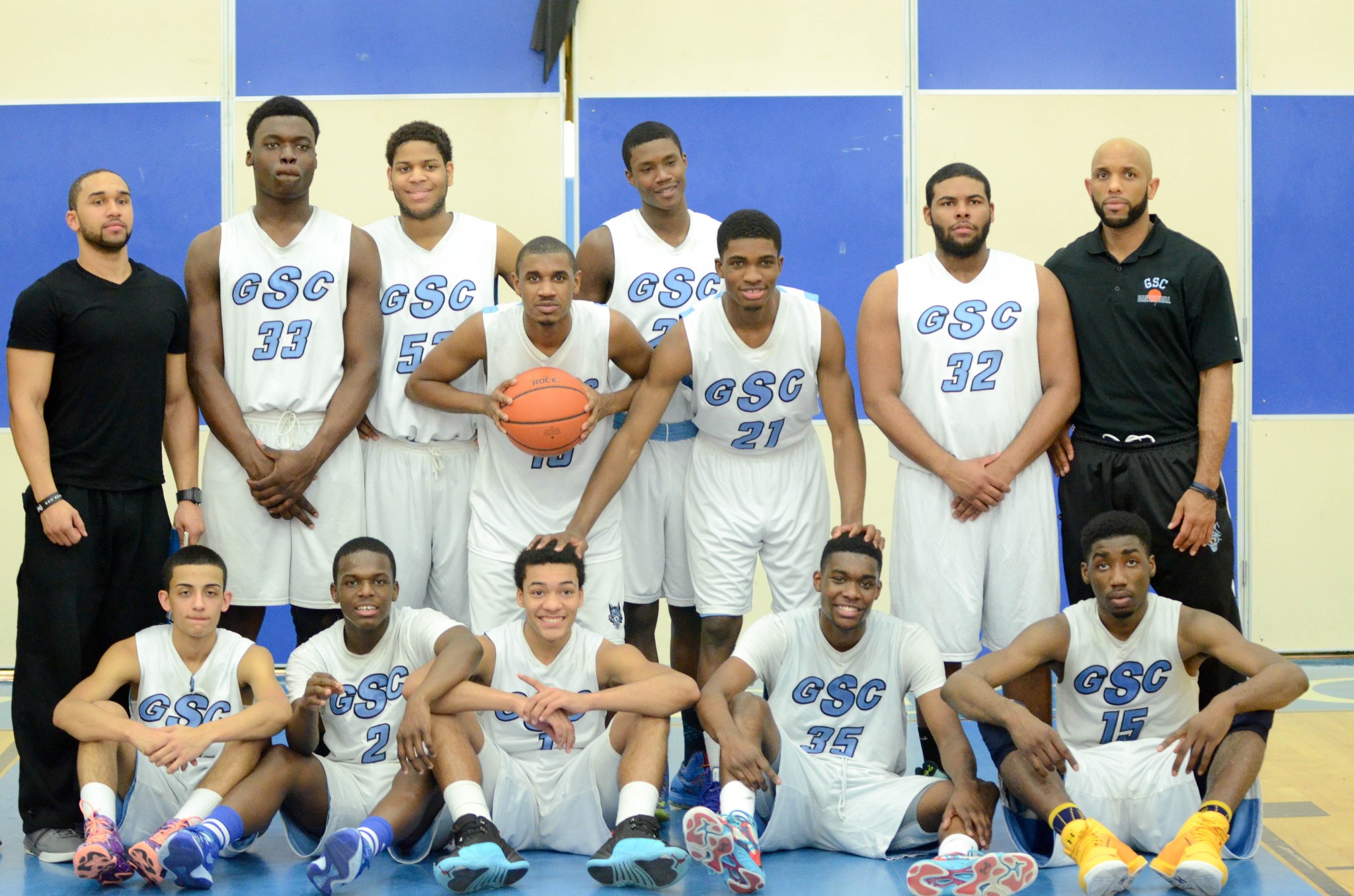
499;367;590;458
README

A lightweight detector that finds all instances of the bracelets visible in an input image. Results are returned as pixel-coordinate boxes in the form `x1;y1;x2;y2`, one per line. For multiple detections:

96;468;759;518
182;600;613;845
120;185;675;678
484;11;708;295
35;492;63;514
1189;481;1220;501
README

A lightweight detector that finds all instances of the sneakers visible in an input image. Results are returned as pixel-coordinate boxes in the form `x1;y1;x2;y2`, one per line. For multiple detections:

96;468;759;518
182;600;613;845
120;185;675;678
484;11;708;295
906;849;1038;896
1060;817;1148;896
126;816;204;884
72;800;134;887
433;813;530;893
586;815;692;890
697;766;721;815
23;828;85;863
682;805;765;894
1150;812;1228;896
668;750;713;810
306;828;372;896
158;824;220;890
654;772;671;821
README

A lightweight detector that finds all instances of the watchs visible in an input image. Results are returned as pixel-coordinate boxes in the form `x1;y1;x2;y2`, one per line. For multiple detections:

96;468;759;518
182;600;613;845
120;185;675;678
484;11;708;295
176;487;202;506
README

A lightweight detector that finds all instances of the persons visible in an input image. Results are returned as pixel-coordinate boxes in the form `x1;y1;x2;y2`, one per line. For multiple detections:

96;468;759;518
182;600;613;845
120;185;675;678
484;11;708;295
157;537;484;896
1045;137;1248;800
528;208;887;814
183;95;384;755
357;121;524;634
857;162;1082;867
401;538;701;894
681;530;1039;896
940;510;1310;896
404;236;655;645
570;122;721;822
53;544;293;888
7;167;206;863
